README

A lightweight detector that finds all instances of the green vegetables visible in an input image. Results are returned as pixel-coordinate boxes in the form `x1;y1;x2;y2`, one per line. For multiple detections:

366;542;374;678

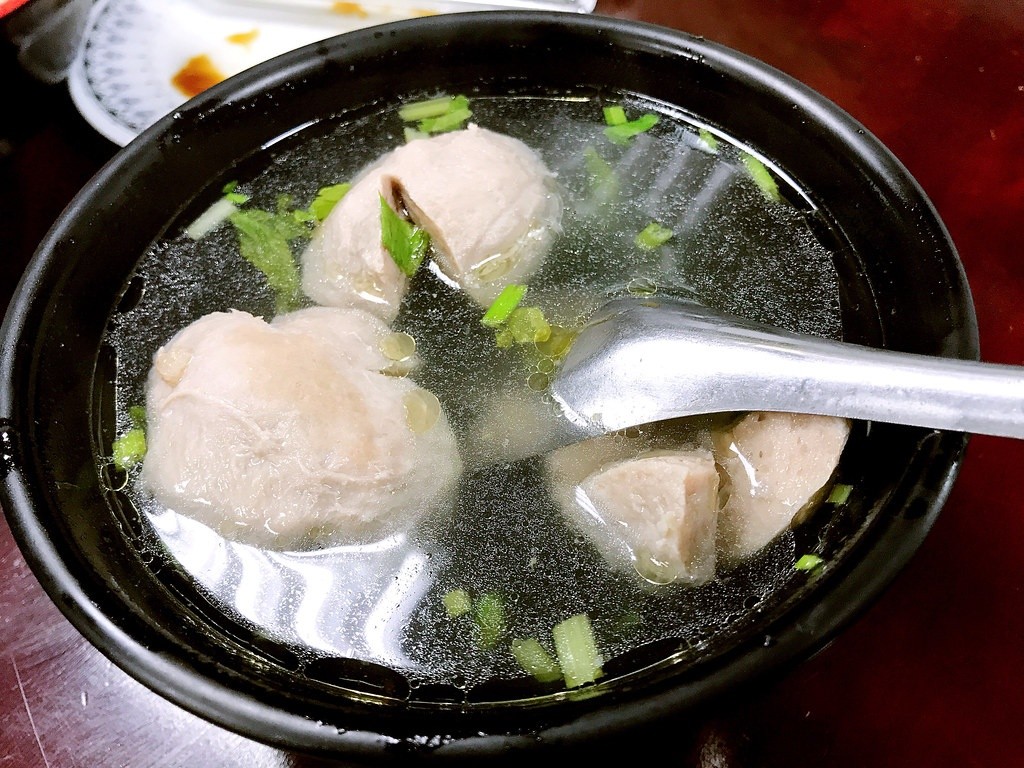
109;94;853;693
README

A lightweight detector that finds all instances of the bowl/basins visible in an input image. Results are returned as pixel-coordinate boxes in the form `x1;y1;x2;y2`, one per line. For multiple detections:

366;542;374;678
0;10;1024;768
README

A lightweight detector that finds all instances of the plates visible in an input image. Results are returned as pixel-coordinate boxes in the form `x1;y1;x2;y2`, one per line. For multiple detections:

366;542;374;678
68;0;597;151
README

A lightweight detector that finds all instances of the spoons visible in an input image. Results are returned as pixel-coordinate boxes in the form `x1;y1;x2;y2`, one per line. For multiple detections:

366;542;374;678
470;298;1024;472
